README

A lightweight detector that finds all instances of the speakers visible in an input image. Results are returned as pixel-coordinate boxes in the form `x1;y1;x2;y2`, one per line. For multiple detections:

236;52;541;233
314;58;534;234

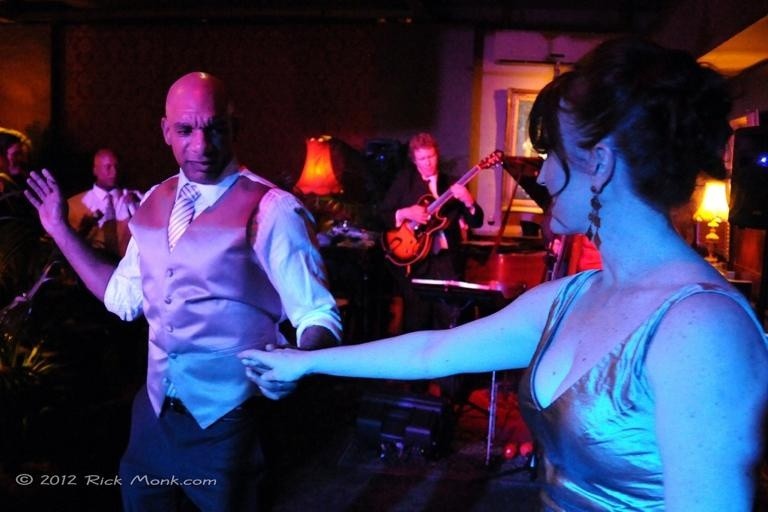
354;392;456;463
726;125;767;232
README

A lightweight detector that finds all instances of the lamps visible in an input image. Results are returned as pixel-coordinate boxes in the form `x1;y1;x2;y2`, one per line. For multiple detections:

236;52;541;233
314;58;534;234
290;138;344;217
691;180;729;263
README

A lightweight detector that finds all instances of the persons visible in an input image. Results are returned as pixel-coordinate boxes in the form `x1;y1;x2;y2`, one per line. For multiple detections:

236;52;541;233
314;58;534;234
79;146;145;263
22;70;343;512
236;32;767;511
381;132;486;408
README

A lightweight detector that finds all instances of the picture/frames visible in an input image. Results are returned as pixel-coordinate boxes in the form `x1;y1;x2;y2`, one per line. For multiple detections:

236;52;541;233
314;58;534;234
500;85;548;217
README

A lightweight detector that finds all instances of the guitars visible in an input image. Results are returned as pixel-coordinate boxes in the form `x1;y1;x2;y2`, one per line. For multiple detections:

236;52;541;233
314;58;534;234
380;147;505;267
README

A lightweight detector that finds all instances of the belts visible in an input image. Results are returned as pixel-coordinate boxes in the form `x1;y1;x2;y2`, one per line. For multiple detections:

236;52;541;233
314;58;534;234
161;394;245;423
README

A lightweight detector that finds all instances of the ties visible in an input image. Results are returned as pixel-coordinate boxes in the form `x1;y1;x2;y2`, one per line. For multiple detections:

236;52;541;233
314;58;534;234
103;193;119;268
167;183;201;255
